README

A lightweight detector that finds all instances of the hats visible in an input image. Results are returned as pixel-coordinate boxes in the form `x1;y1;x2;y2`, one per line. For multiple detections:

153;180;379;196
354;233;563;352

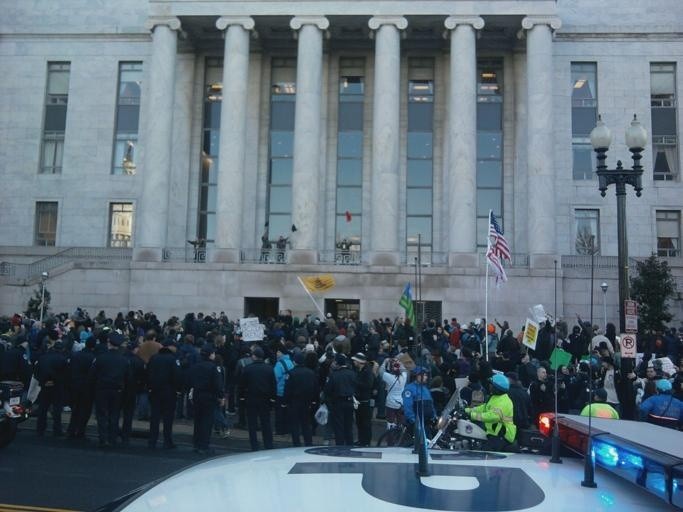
202;345;220;356
487;325;495;333
253;349;264;357
292;352;304;363
594;342;608;351
278;345;288;355
333;355;347;367
351;352;367;364
594;389;607;398
602;357;615;365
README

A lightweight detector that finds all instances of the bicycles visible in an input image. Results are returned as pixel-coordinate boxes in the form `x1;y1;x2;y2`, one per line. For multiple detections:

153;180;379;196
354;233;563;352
370;411;418;449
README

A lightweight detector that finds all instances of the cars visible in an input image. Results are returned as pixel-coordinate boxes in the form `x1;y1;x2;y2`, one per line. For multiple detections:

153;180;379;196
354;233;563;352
103;410;683;512
0;379;27;446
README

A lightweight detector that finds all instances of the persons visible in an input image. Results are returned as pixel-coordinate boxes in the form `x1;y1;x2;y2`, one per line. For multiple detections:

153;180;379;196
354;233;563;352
335;238;353;265
259;229;273;264
275;235;291;264
0;308;683;459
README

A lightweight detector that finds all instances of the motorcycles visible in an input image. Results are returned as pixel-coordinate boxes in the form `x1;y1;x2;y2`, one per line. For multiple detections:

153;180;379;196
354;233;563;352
378;410;551;455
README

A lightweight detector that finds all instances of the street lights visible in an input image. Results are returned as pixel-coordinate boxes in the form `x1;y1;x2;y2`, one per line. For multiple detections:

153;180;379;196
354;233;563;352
590;113;647;421
600;282;609;334
39;271;49;325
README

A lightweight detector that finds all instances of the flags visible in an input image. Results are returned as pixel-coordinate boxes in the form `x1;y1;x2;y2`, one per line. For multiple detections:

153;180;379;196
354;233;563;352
300;272;337;294
397;280;417;328
489;209;512;262
264;220;270;227
485;242;508;289
291;224;298;232
345;209;351;223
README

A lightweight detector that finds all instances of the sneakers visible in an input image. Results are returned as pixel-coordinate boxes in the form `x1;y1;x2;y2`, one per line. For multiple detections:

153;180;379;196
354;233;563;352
55;426;86;439
224;430;231;440
199;447;214;454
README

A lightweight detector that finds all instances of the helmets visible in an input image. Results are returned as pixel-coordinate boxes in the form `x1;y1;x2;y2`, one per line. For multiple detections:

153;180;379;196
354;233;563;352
655;378;672;391
412;367;427;375
489;374;509;392
